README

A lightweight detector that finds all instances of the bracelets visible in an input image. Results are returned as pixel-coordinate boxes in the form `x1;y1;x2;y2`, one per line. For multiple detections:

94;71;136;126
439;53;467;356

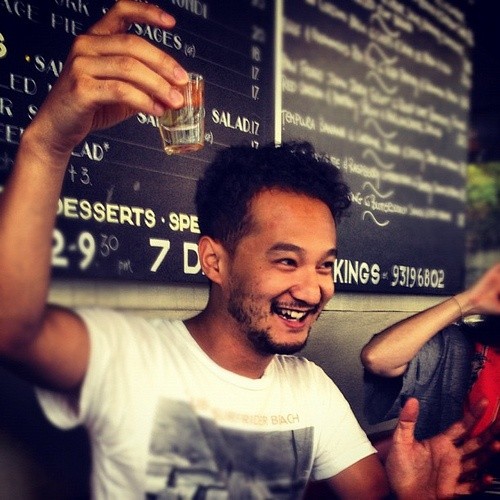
453;297;464;318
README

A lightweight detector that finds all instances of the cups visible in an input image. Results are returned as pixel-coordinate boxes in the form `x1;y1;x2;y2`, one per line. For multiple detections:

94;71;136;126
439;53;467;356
156;73;205;155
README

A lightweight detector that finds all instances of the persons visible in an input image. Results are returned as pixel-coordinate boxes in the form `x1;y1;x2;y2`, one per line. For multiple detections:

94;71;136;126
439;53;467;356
361;260;499;499
0;0;500;500
